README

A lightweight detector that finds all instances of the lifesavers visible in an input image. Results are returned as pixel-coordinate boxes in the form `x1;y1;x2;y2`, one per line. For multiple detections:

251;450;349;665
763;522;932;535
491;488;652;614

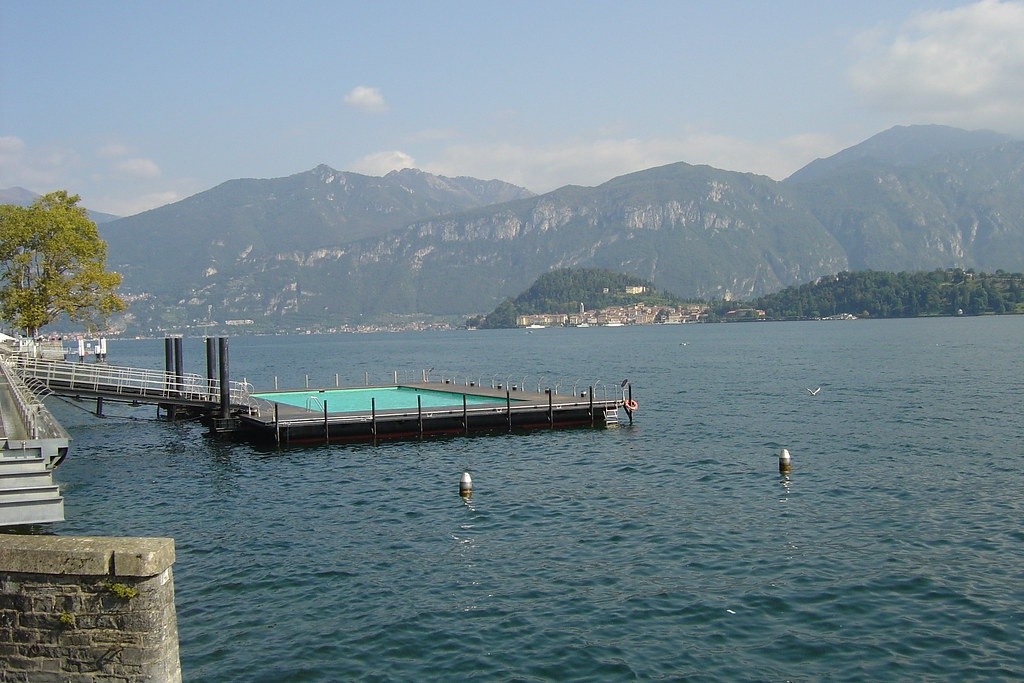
625;399;638;410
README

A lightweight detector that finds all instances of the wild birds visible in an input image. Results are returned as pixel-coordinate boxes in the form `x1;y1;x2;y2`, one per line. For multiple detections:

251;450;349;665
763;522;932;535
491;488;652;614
807;387;821;396
